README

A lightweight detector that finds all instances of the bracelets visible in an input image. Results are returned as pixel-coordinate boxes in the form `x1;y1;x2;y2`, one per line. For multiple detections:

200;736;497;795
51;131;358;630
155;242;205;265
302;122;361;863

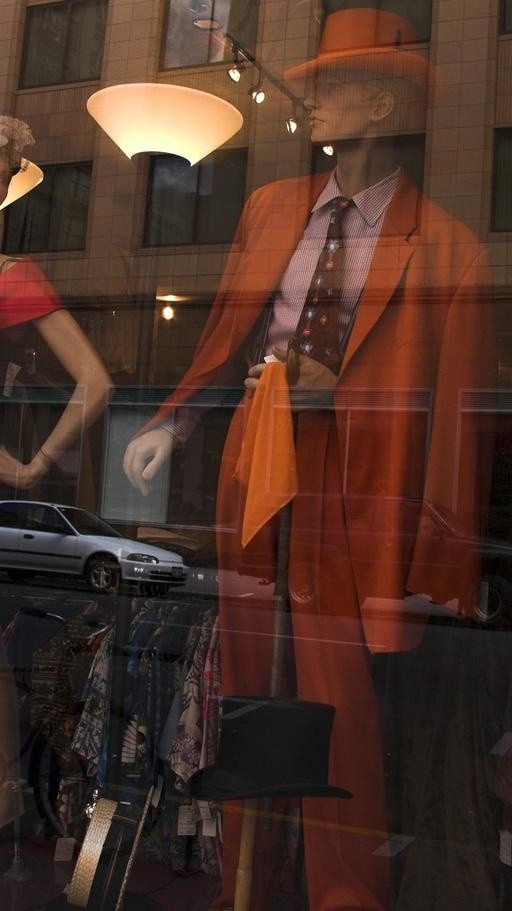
38;446;56;468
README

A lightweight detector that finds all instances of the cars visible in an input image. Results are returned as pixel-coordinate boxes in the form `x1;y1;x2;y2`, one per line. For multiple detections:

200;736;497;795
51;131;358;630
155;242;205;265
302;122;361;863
0;500;190;598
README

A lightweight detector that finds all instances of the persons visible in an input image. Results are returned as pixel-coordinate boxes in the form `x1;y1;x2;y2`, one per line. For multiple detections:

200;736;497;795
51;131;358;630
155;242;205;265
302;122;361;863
0;112;116;491
122;10;488;911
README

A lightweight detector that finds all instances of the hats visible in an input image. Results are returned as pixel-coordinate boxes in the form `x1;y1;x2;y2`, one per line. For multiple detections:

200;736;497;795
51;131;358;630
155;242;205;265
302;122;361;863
186;694;356;803
280;7;446;134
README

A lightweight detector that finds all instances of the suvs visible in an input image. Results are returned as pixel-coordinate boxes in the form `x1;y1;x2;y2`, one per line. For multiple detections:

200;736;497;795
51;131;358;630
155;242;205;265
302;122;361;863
237;479;512;623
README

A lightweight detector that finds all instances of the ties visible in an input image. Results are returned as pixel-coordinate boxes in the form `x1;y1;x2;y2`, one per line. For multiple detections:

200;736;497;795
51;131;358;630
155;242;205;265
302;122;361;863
285;196;356;378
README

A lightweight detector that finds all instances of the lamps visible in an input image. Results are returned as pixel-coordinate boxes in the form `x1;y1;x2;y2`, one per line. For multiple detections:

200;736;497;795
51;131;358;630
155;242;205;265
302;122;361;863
188;3;301;137
86;84;245;178
